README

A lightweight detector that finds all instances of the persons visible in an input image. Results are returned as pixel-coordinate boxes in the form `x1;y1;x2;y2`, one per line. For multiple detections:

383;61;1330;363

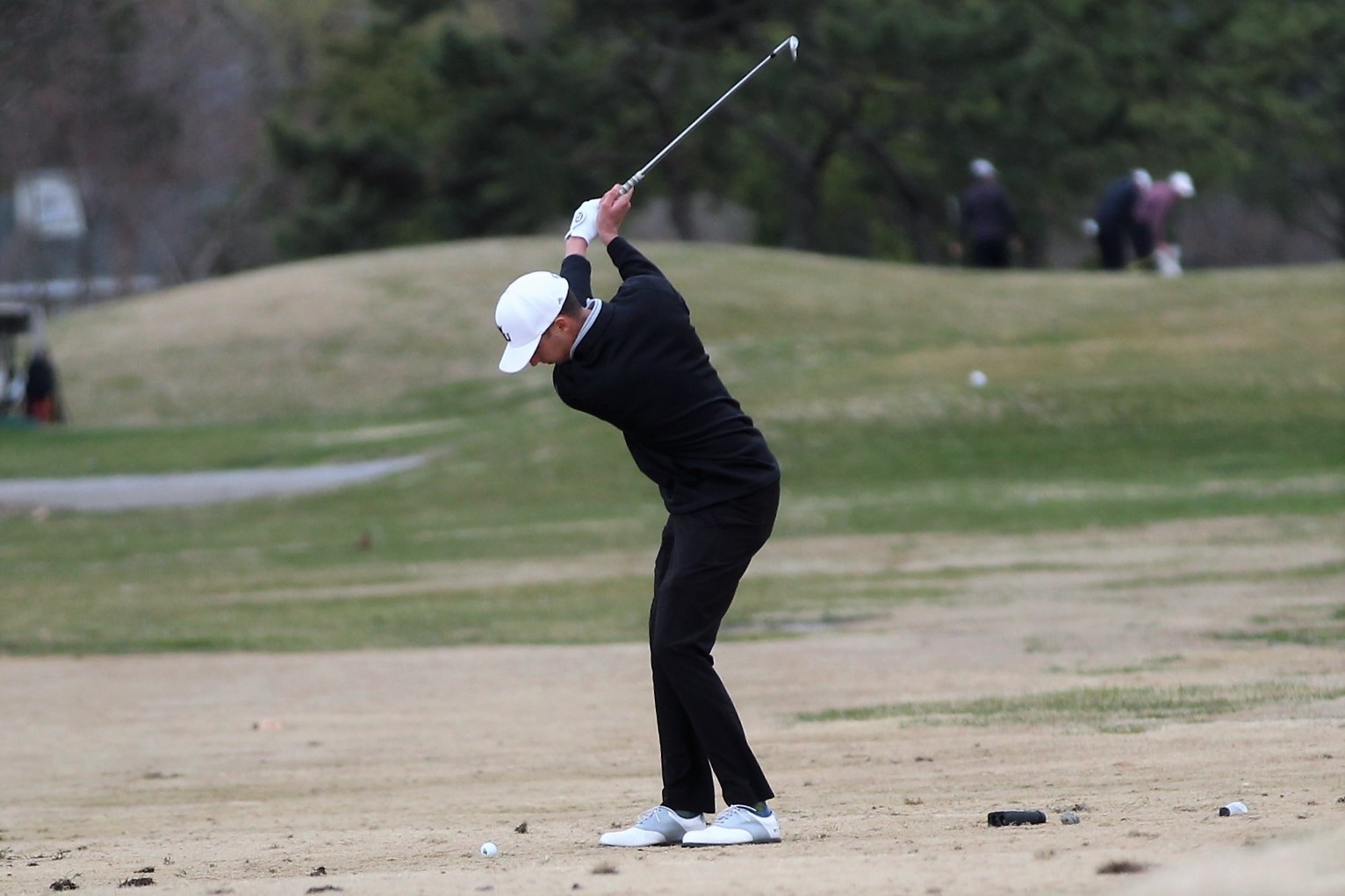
1085;170;1201;279
493;181;800;848
952;160;1028;273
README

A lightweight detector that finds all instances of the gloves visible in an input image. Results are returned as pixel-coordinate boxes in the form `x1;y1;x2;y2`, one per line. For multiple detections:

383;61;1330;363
564;198;599;247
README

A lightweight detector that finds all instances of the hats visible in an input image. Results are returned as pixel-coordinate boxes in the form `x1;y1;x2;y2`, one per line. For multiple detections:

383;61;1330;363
1174;172;1196;197
967;156;998;177
495;271;568;373
1135;171;1154;193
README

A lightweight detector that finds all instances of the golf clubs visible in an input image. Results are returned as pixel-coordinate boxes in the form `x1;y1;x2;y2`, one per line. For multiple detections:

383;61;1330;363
616;32;799;197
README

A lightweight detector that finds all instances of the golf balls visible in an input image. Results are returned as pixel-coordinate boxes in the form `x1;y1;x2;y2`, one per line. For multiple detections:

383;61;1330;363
480;842;499;858
968;370;990;389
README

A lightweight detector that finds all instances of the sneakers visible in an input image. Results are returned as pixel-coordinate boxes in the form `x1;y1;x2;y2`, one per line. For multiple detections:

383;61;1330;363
682;804;782;847
601;807;706;846
